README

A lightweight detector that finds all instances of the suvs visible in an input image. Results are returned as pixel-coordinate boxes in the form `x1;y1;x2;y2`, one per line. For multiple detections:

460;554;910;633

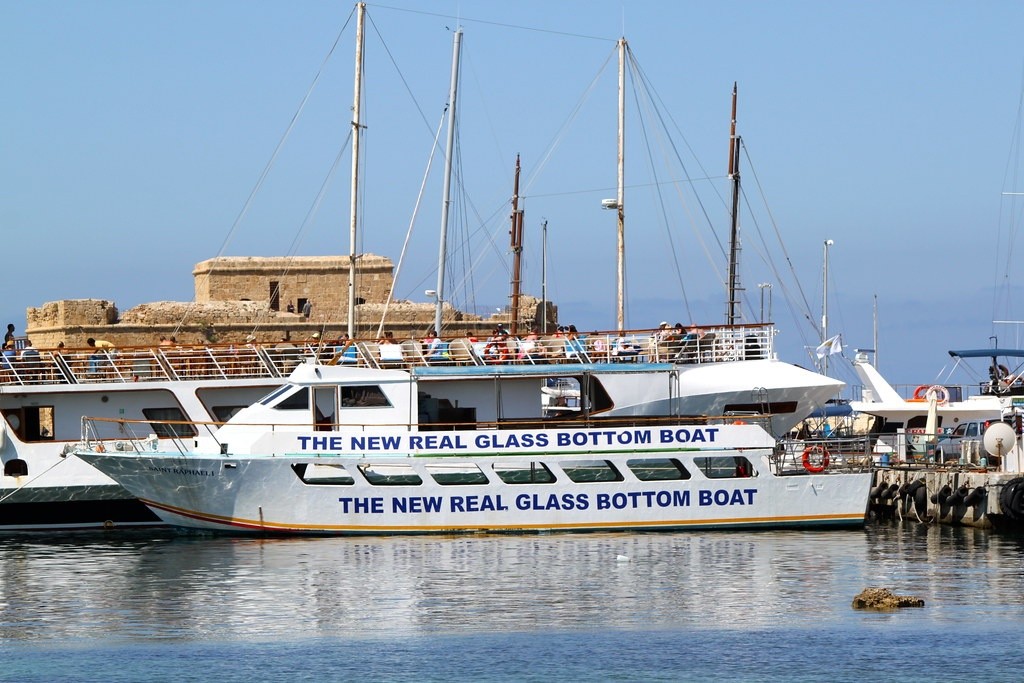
934;418;1012;466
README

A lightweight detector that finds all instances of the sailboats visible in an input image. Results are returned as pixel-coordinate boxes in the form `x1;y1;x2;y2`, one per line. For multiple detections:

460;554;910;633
0;0;846;536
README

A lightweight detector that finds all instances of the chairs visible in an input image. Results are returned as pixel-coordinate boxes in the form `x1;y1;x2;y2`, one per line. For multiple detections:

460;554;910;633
131;353;153;383
166;349;187;381
357;334;676;369
280;349;301;372
686;333;700;350
1;350;16;382
21;350;46;385
235;351;253;379
211;352;233;380
699;333;716;363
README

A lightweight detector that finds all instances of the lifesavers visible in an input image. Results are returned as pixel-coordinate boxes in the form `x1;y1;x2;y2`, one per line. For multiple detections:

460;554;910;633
999;477;1023;518
731;420;747;425
484;340;508;364
926;385;951;402
801;446;830;471
913;385;930;401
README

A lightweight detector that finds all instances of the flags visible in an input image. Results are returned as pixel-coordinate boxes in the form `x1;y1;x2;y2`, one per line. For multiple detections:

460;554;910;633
816;334;842;360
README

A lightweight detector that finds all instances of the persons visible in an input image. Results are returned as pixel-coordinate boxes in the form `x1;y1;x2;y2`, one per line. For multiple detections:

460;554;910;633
287;302;294;313
467;323;637;365
378;331;443;367
275;334;299;374
245;335;257;348
159;335;183;352
0;323;40;384
48;340;68;383
304;332;351;359
649;321;705;364
87;338;117;378
302;299;312;321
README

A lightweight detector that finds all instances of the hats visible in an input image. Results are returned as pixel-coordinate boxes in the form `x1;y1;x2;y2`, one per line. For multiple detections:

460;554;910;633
246;336;255;343
312;333;320;339
5;341;16;348
497;323;503;327
660;322;666;326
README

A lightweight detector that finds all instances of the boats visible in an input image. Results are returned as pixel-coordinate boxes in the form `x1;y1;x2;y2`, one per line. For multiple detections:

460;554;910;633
74;358;873;537
